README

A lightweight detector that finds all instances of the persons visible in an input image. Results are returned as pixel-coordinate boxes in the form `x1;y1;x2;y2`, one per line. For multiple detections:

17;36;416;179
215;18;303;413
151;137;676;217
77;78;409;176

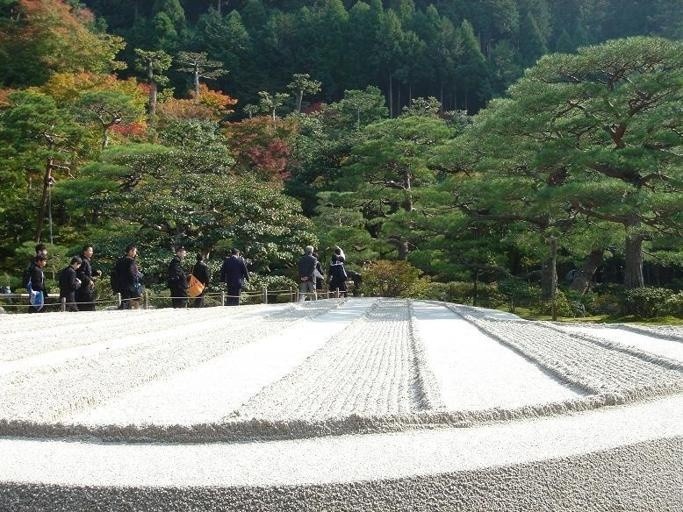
327;244;347;297
220;247;250;305
27;244;48;279
295;244;324;301
116;244;140;310
190;252;213;308
73;244;103;311
312;252;323;290
28;253;46;313
164;247;191;308
58;256;82;311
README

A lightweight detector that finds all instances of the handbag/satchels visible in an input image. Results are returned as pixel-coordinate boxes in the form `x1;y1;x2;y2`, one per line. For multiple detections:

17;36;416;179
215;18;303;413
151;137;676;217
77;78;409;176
27;285;45;310
130;286;140;301
187;274;203;297
